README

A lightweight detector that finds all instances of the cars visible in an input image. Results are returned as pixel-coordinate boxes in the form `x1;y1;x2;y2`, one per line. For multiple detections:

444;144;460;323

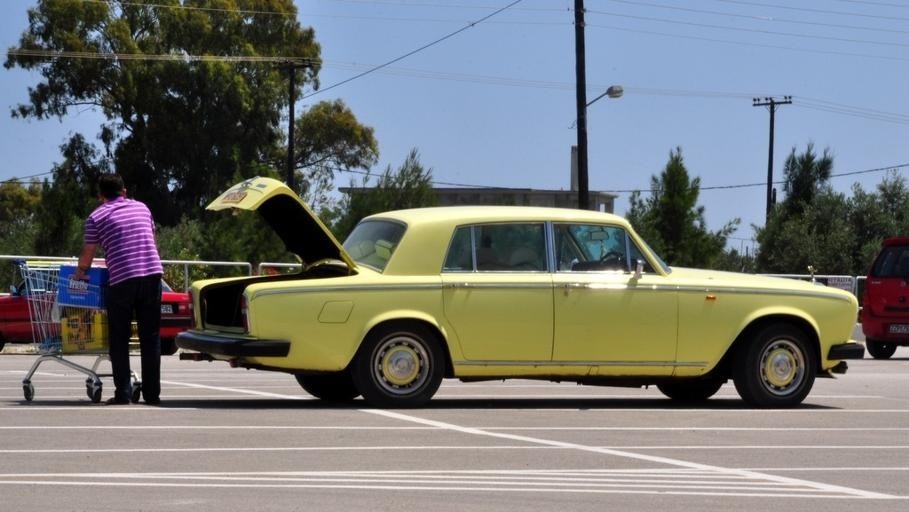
174;175;867;411
0;262;192;357
861;236;909;359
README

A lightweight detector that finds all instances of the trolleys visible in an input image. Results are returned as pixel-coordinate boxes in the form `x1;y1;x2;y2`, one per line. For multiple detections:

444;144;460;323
18;263;141;402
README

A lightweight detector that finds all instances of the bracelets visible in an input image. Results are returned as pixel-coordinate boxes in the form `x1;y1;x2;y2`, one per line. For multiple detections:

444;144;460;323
77;265;89;273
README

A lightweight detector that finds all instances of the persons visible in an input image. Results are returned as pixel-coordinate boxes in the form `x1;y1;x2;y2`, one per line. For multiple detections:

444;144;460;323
71;172;165;407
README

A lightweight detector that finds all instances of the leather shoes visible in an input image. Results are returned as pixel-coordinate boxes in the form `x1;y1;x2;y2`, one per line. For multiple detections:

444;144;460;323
107;392;162;405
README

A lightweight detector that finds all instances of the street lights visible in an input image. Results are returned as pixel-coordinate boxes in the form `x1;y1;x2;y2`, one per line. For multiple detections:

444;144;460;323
574;0;623;210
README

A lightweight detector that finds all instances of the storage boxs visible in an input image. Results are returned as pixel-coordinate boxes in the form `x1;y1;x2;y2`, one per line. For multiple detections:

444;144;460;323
60;309;108;352
57;264;109;309
